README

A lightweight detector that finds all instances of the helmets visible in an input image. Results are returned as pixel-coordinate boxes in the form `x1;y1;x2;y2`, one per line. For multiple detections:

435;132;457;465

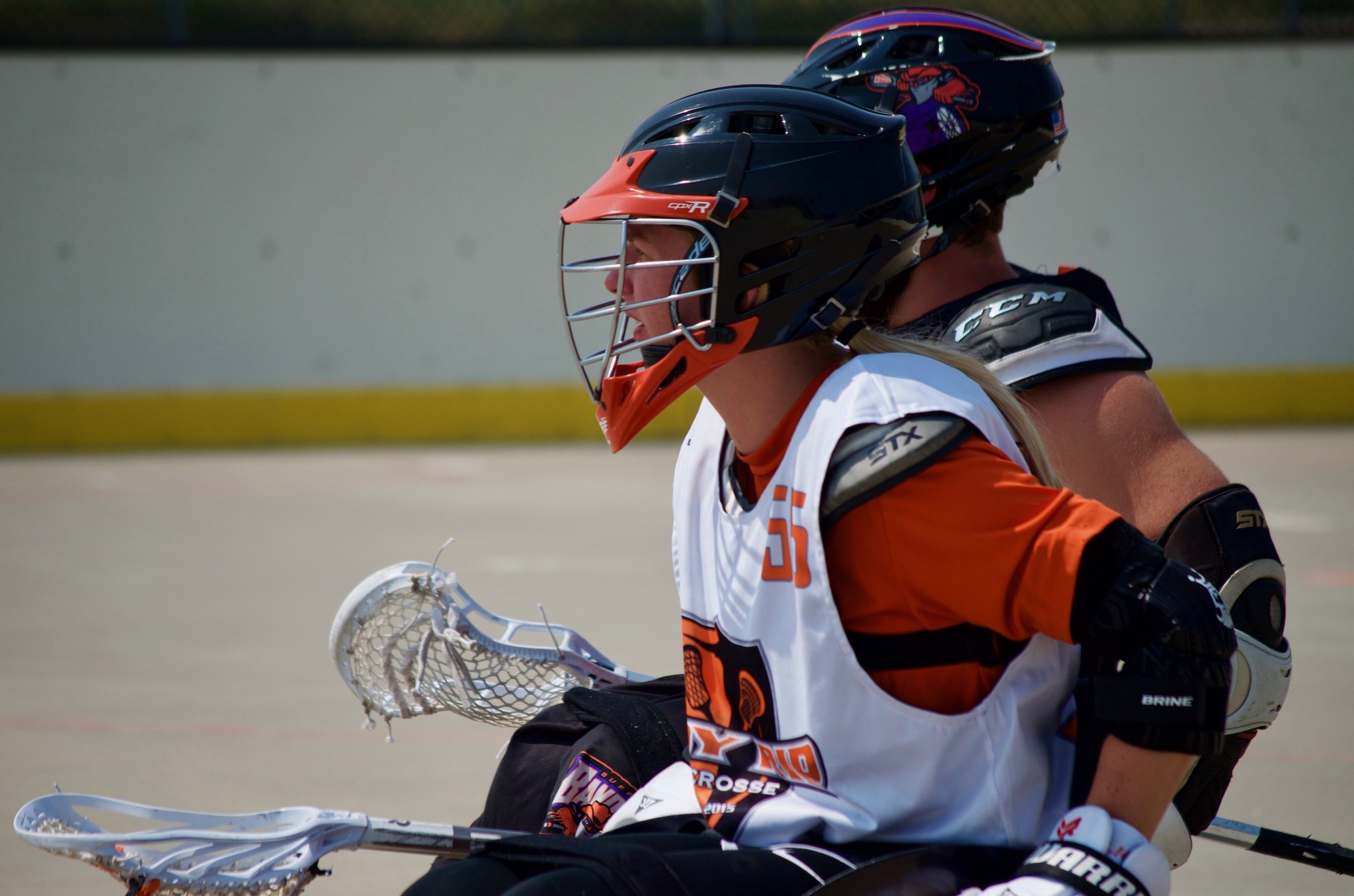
782;8;1068;260
560;84;945;462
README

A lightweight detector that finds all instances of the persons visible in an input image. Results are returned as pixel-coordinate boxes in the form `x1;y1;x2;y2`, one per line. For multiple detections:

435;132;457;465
477;1;1294;869
401;82;1237;896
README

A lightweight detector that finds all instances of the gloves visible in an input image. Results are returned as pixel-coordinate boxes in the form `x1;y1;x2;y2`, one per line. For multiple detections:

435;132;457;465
958;807;1170;896
